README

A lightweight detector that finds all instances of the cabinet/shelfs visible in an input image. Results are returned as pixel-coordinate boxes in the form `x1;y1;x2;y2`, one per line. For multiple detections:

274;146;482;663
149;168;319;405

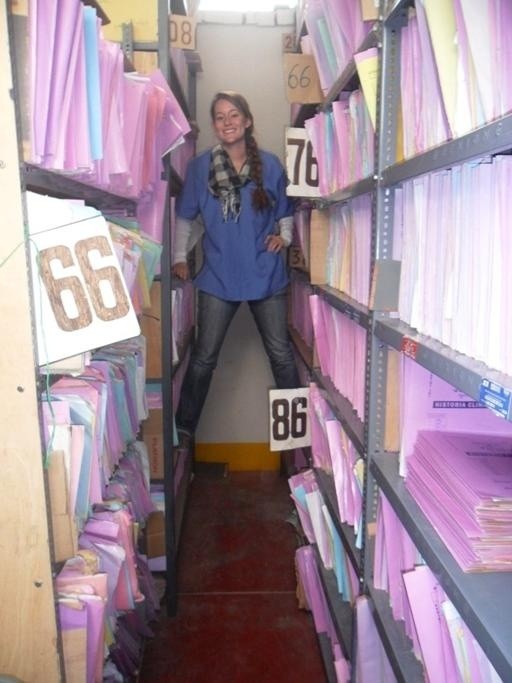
0;0;201;683
285;0;511;683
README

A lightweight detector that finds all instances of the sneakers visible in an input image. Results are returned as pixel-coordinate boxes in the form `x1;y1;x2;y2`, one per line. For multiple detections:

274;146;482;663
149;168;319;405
174;431;192;453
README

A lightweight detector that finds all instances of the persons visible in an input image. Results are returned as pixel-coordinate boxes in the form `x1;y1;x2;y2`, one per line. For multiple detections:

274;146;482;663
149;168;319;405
176;90;303;453
